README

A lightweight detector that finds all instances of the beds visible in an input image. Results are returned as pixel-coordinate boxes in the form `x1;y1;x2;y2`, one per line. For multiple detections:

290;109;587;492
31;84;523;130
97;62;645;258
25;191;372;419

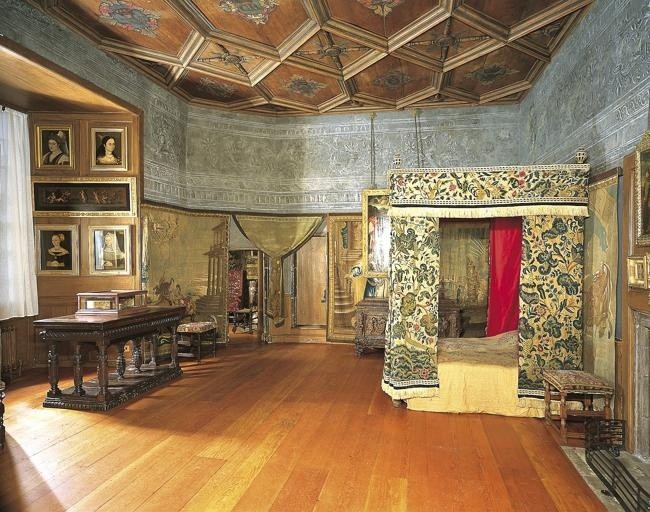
404;329;592;420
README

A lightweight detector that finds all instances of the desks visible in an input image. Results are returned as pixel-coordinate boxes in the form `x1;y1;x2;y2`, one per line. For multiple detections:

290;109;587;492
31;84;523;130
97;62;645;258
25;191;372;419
31;303;187;414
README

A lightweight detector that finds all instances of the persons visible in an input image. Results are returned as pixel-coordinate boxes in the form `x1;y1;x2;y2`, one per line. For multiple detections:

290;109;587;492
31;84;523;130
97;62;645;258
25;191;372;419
92;134;123;165
44;231;71;269
101;231;126;261
41;128;70;165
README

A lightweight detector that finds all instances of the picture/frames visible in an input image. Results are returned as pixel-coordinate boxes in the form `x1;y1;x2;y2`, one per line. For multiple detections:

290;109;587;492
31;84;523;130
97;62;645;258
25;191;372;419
23;118;138;278
631;129;650;248
626;255;648;291
361;189;392;279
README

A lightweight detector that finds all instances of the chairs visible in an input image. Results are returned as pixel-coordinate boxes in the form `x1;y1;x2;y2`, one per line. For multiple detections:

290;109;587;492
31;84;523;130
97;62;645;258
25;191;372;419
233;295;260;333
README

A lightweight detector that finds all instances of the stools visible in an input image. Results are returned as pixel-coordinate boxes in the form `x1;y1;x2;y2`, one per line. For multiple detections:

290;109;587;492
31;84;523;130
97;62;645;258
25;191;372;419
539;367;616;444
175;320;219;363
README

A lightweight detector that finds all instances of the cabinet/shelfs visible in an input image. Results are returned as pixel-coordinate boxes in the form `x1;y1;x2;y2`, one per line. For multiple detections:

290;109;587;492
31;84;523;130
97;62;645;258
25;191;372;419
227;269;244;318
351;298;462;358
